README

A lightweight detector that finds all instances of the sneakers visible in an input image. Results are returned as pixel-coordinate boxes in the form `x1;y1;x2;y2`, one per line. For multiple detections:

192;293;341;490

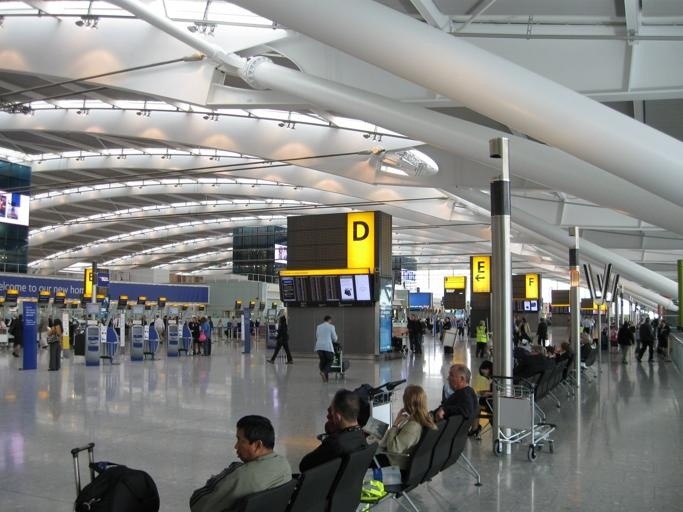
320;370;328;384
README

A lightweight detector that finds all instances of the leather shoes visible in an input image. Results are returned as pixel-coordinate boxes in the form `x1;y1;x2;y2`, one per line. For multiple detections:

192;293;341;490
266;360;274;364
285;361;293;364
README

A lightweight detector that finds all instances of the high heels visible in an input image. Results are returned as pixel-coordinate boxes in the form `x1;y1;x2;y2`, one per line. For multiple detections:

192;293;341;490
467;428;479;437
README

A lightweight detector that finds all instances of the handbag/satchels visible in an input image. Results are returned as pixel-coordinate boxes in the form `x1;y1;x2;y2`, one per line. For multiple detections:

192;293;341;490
74;461;159;512
8;324;15;336
47;326;59;344
199;329;206;342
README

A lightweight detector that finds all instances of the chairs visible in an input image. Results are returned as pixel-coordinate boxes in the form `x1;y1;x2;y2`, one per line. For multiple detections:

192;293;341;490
233;408;481;511
579;339;601;383
470;347;577;437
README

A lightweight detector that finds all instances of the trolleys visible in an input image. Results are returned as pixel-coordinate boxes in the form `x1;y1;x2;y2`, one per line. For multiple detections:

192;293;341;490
328;342;349;379
397;331;408;353
486;375;556;462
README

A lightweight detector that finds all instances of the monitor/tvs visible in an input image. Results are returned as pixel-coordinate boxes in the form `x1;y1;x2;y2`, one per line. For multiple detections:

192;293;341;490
169;326;177;332
133;327;142;335
0;190;30;227
88;328;98;335
274;243;287;264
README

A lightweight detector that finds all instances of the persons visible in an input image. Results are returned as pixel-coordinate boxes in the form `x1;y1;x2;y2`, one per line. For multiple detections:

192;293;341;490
474;360;497;427
514;316;597;360
265;309;294;364
380;381;441;489
299;389;371;512
430;365;482;441
9;313;237;372
408;313;492;357
187;414;291;511
616;316;682;364
314;317;339;381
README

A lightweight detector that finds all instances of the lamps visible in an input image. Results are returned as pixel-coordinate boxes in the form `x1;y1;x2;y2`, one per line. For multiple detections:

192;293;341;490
75;0;102;29
187;2;216;37
